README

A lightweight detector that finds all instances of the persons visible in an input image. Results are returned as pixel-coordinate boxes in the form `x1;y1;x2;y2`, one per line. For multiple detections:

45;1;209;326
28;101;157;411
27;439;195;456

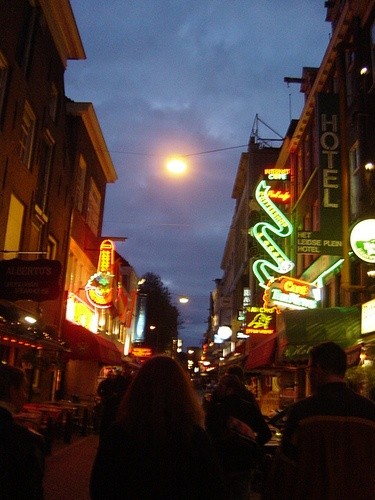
0;340;375;500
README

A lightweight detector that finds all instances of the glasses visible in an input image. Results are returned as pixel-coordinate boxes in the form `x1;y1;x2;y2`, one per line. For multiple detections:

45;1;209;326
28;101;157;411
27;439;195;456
303;365;314;373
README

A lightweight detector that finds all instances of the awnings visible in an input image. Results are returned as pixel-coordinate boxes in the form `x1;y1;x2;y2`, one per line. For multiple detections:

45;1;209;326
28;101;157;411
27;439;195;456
66;324;122;366
245;341;278;369
